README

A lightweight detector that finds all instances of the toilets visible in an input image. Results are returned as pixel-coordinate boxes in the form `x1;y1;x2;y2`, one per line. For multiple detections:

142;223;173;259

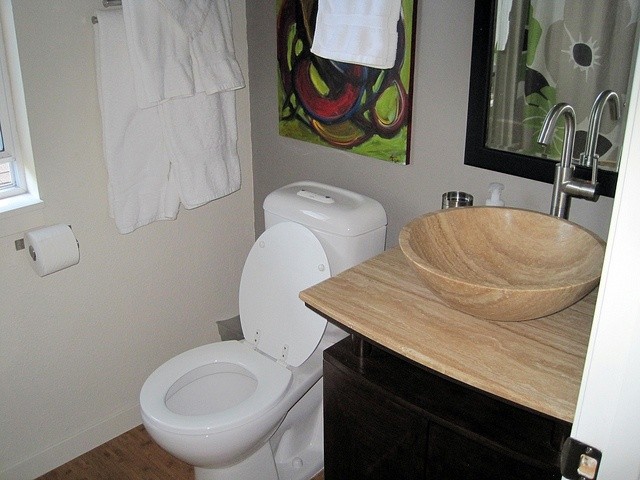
139;180;387;480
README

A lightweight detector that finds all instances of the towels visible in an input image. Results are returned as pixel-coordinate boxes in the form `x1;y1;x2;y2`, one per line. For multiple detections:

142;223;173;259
122;0;247;109
93;10;242;234
310;0;400;70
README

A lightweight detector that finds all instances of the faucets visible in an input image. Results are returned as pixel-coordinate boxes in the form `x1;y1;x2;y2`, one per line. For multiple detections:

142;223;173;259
538;102;577;221
581;90;622;169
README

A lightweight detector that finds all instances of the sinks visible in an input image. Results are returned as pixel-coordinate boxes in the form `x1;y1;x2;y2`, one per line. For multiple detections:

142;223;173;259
398;205;608;324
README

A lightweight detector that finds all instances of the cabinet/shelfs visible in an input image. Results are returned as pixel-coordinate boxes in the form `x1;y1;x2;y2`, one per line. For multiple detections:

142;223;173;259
322;336;567;480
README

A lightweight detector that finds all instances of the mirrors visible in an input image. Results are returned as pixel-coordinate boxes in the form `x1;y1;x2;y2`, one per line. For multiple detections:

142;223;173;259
463;0;639;201
